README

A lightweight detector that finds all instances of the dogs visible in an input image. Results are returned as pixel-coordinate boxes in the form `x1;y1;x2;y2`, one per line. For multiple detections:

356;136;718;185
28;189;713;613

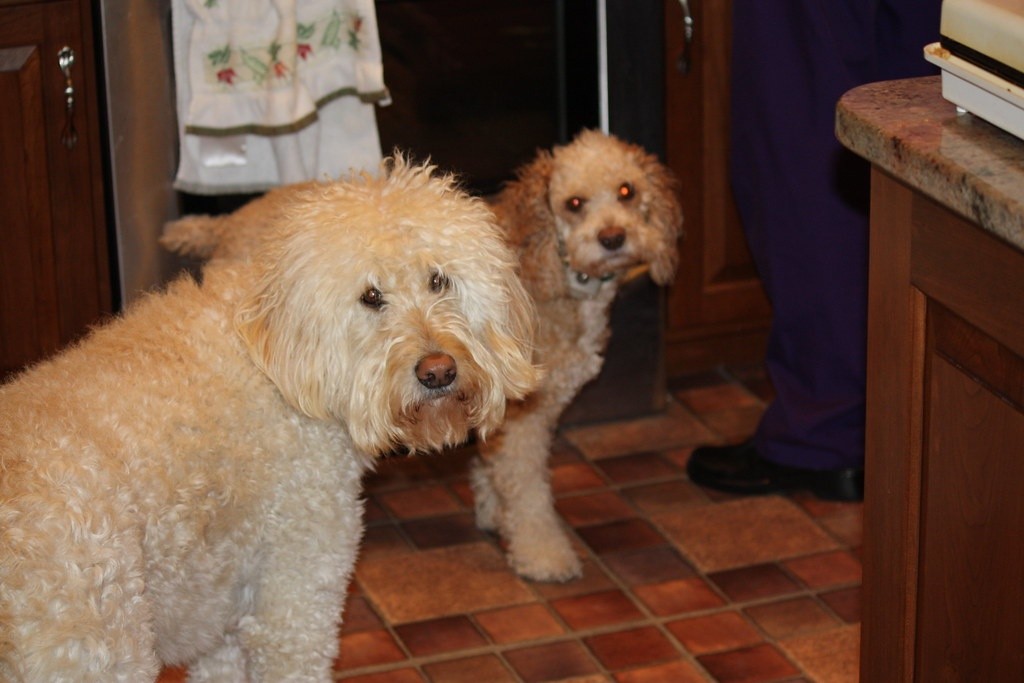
0;147;551;683
158;129;684;583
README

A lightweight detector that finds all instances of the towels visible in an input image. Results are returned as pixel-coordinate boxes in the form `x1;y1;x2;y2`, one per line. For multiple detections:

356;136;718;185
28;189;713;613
167;1;394;199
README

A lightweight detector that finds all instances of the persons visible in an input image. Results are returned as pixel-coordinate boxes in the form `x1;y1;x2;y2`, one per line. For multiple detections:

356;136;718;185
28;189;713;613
683;0;942;504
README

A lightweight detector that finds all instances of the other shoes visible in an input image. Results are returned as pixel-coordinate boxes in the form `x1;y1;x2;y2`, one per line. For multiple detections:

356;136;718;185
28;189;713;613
688;438;863;502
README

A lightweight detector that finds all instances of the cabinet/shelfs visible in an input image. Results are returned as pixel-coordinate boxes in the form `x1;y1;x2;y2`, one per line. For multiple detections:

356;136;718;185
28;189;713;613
0;1;117;385
